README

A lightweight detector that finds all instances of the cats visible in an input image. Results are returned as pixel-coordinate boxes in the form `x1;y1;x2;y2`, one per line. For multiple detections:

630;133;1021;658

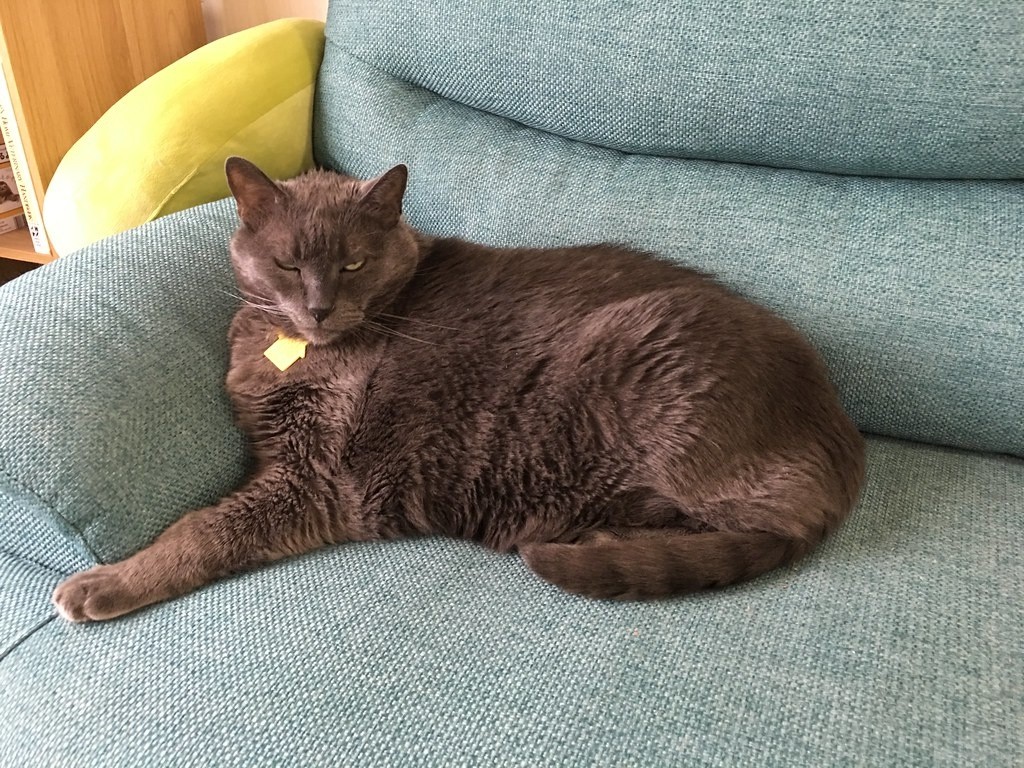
53;157;867;623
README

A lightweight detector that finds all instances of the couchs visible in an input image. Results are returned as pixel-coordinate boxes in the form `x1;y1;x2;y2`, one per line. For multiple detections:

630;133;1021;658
0;0;1024;768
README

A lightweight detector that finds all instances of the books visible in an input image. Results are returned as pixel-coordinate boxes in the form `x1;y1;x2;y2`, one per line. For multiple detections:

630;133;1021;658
0;63;53;255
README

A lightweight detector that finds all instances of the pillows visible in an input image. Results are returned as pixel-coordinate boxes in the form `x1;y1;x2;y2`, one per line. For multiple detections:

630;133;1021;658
41;17;329;257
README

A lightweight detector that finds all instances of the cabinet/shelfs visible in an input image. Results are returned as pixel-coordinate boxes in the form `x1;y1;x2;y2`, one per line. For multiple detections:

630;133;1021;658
0;0;207;267
